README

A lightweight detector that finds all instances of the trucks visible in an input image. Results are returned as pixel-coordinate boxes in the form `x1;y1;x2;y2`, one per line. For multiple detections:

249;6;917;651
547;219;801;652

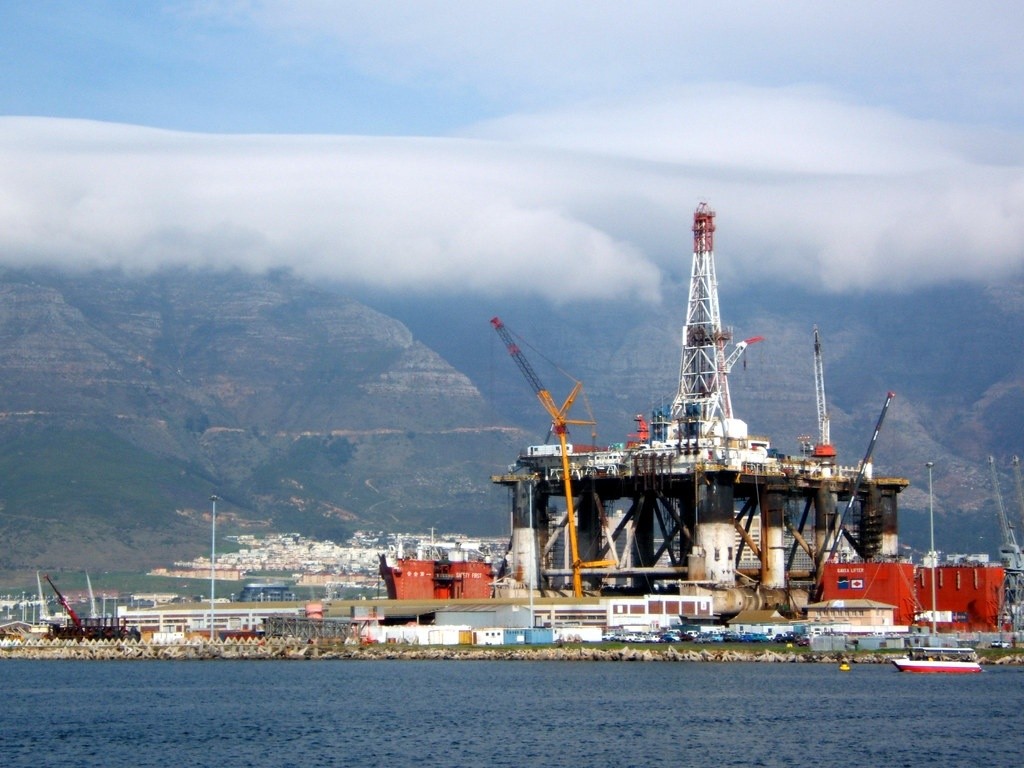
946;552;989;566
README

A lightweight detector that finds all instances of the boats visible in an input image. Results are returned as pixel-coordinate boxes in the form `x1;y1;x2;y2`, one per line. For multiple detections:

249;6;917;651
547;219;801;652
902;645;978;661
889;657;983;675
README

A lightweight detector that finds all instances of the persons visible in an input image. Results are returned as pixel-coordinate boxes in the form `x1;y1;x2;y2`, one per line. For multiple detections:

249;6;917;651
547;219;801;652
923;651;928;661
966;655;970;661
907;649;922;661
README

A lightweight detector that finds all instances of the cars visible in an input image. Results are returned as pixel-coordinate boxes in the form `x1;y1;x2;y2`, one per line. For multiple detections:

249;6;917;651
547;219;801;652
602;628;840;648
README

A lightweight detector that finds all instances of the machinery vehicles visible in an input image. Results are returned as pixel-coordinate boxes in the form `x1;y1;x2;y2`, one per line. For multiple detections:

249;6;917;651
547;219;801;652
37;574;130;638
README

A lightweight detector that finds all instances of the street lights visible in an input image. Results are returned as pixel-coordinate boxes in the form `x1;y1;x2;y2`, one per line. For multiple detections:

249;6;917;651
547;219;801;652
922;460;943;634
208;493;222;642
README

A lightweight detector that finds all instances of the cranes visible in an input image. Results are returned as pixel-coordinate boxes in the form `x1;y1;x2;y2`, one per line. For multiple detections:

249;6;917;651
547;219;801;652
986;455;1021;572
1009;455;1023;559
